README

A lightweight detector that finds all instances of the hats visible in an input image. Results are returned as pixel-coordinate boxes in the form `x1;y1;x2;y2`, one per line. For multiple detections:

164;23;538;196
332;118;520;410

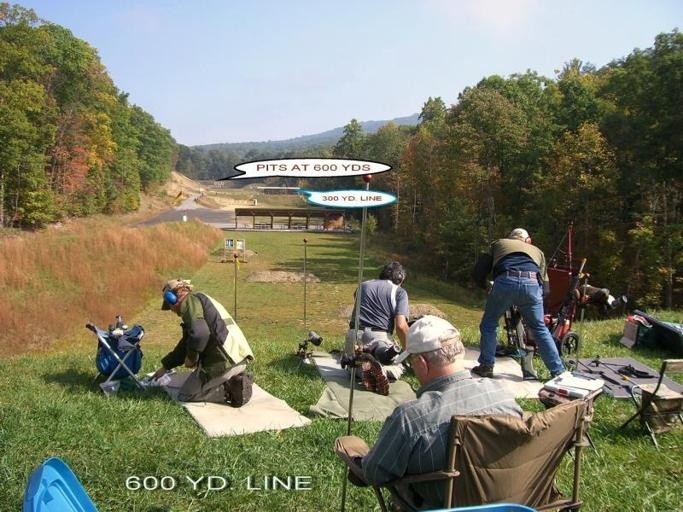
394;315;460;363
161;279;182;311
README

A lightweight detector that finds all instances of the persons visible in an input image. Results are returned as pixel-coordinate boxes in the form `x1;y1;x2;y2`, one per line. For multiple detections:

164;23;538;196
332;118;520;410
345;261;410;380
146;278;254;407
362;315;525;510
471;228;565;378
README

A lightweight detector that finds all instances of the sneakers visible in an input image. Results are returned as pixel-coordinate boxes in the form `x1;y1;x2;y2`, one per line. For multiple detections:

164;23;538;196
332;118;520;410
471;363;493;378
361;359;389;396
226;373;252;408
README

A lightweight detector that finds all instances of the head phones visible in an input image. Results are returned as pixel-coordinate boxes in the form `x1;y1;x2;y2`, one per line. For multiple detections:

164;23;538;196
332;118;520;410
525;236;531;244
162;285;178;305
392;261;404;284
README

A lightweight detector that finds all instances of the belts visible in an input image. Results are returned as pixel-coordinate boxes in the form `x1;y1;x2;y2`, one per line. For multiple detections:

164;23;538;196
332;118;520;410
502;271;536;278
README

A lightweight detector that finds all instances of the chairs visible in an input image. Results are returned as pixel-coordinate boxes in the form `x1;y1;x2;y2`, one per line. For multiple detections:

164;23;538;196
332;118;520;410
618;357;683;453
633;310;682;353
331;388;606;511
